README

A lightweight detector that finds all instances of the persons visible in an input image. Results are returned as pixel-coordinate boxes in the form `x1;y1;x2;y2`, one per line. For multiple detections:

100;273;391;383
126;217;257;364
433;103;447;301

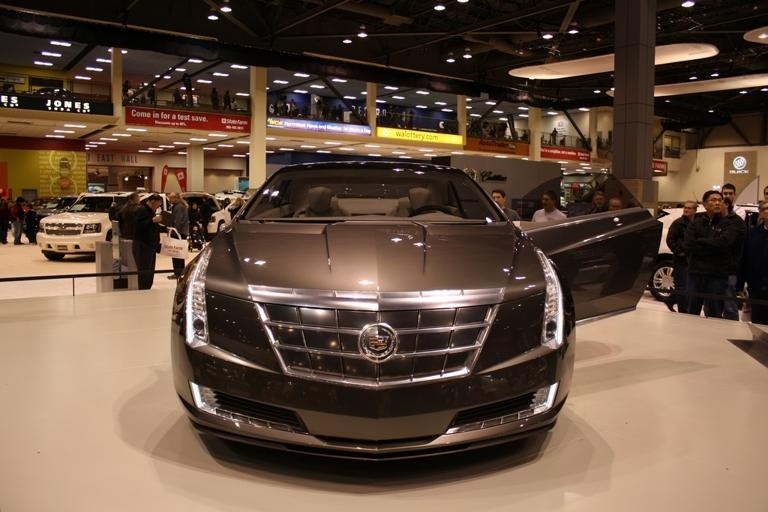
0;188;37;246
531;191;567;223
491;188;523;221
584;191;608;214
608;197;625;212
107;192;246;290
124;71;559;145
665;183;768;325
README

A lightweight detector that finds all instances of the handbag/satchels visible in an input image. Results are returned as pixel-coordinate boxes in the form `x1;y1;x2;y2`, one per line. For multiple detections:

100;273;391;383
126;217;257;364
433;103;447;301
158;235;190;260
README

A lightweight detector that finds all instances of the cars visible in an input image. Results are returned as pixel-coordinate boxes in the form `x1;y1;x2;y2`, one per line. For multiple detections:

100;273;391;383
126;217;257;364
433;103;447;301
23;196;78;241
172;161;663;462
654;204;758;302
174;191;248;238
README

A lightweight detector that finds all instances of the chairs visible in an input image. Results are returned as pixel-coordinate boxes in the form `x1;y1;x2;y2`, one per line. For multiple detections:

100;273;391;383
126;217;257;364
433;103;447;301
292;187;352;220
386;187;439;217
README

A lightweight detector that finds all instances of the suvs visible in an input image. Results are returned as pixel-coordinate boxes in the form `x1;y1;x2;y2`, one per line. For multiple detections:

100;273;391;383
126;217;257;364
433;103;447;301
36;191;173;259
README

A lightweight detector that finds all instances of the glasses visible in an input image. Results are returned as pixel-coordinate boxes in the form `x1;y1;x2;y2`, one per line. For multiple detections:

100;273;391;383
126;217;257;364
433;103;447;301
707;198;724;204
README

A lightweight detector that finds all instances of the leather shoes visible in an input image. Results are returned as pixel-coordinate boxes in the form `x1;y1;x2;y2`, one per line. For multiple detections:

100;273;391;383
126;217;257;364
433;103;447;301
167;274;177;279
